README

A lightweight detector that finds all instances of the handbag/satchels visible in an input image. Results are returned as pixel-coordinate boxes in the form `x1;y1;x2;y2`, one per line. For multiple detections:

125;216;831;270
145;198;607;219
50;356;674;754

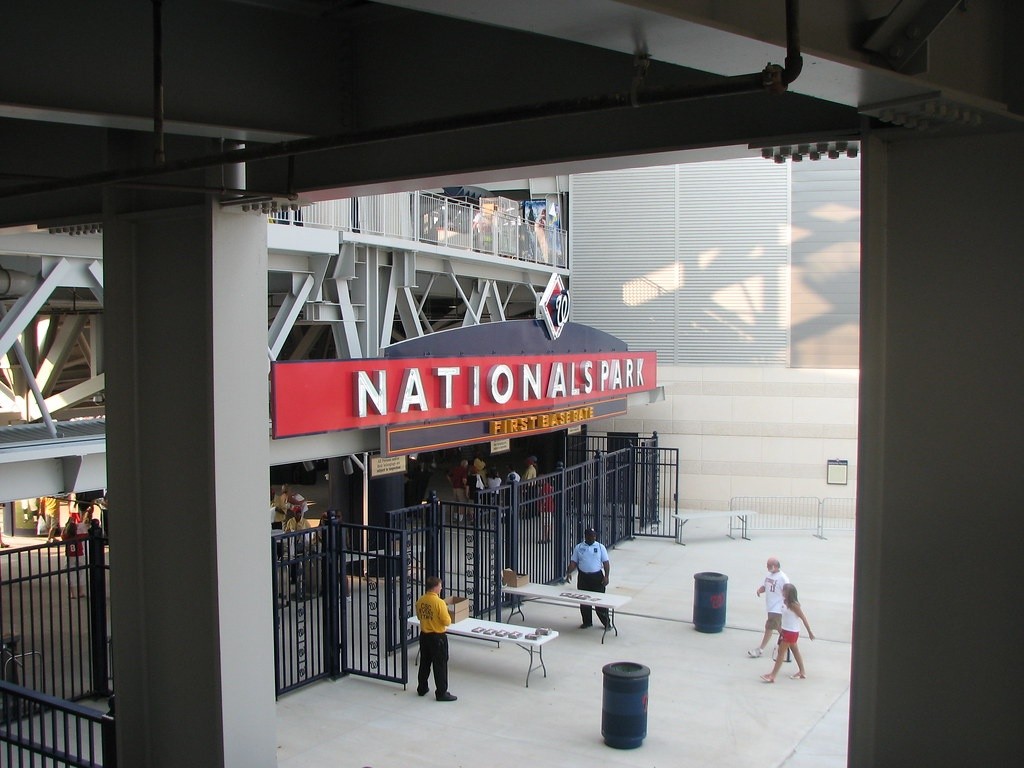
773;636;791;664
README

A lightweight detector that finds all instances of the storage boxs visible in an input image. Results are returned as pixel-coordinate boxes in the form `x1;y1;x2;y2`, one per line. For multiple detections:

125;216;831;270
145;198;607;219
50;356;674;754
502;568;529;588
444;596;469;624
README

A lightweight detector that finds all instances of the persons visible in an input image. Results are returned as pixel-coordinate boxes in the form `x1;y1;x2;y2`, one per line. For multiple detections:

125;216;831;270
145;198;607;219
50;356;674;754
43;497;61;544
287;492;309;518
747;557;793;663
486;472;503;508
413;577;458;702
61;516;89;599
280;483;289;504
270;488;286;561
466;458;486;519
520;456;539;519
536;473;554;545
505;466;520;483
67;494;81;523
565;528;611;631
334;511;351;603
447;460;470;522
283;506;312;584
761;583;815;682
311;513;327;595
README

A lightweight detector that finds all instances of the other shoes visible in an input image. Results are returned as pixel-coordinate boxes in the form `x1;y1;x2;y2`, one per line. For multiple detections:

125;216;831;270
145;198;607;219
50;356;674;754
538;538;551;544
605;624;611;631
759;673;775;682
453;512;464;522
291;579;297;584
418;688;429;696
339;592;350;598
436;691;457;701
748;648;764;658
580;623;592;628
790;672;806;679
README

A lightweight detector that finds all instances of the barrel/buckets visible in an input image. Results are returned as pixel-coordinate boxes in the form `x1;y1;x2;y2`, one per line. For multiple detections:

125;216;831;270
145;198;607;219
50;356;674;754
601;661;650;750
693;572;728;633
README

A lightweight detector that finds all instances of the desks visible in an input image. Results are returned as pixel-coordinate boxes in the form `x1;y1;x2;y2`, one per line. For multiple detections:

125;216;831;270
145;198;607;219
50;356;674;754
408;615;559;688
671;509;757;546
501;583;631;645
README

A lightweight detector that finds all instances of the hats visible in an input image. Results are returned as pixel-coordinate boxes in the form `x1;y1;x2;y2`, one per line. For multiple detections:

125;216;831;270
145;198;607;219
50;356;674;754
528;455;537;461
292;506;301;512
585;528;598;536
